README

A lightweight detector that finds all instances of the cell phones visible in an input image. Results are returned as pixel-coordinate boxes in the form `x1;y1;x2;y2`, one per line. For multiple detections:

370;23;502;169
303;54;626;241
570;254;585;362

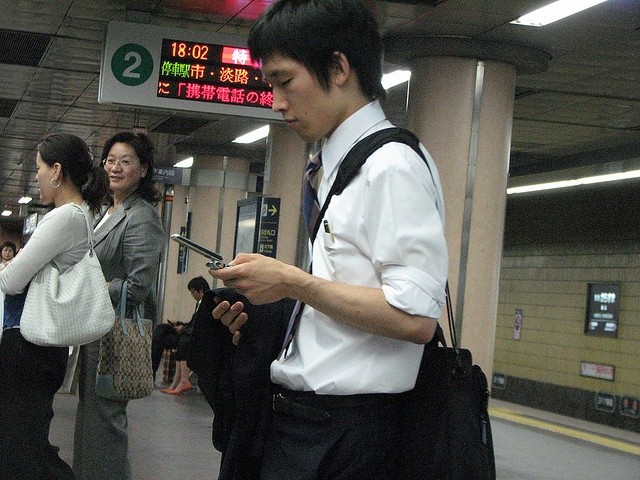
170;234;231;270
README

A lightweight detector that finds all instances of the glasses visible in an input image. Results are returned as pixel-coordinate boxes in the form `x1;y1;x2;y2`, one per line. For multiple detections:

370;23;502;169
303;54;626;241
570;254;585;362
102;158;144;169
190;291;198;295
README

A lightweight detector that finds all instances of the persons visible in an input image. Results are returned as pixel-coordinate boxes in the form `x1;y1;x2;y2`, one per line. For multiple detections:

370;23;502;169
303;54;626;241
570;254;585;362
161;335;193;396
74;128;166;479
206;1;449;480
1;135;95;480
1;237;16;267
148;276;212;383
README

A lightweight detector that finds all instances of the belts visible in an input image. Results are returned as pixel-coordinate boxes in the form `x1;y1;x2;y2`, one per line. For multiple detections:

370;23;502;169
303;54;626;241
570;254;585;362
3;325;19;331
271;392;413;423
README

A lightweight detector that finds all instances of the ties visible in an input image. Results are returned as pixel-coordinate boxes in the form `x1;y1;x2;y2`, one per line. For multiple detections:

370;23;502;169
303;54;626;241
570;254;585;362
275;150;323;357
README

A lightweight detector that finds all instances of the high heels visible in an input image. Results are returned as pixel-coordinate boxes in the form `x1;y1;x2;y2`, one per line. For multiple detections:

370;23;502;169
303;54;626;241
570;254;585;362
160;387;174;394
165;383;193;395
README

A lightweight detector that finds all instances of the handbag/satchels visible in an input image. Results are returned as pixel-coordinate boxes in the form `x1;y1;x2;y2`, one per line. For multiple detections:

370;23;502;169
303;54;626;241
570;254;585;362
20;202;115;347
95;281;154;401
163;348;176;383
413;347;496;479
